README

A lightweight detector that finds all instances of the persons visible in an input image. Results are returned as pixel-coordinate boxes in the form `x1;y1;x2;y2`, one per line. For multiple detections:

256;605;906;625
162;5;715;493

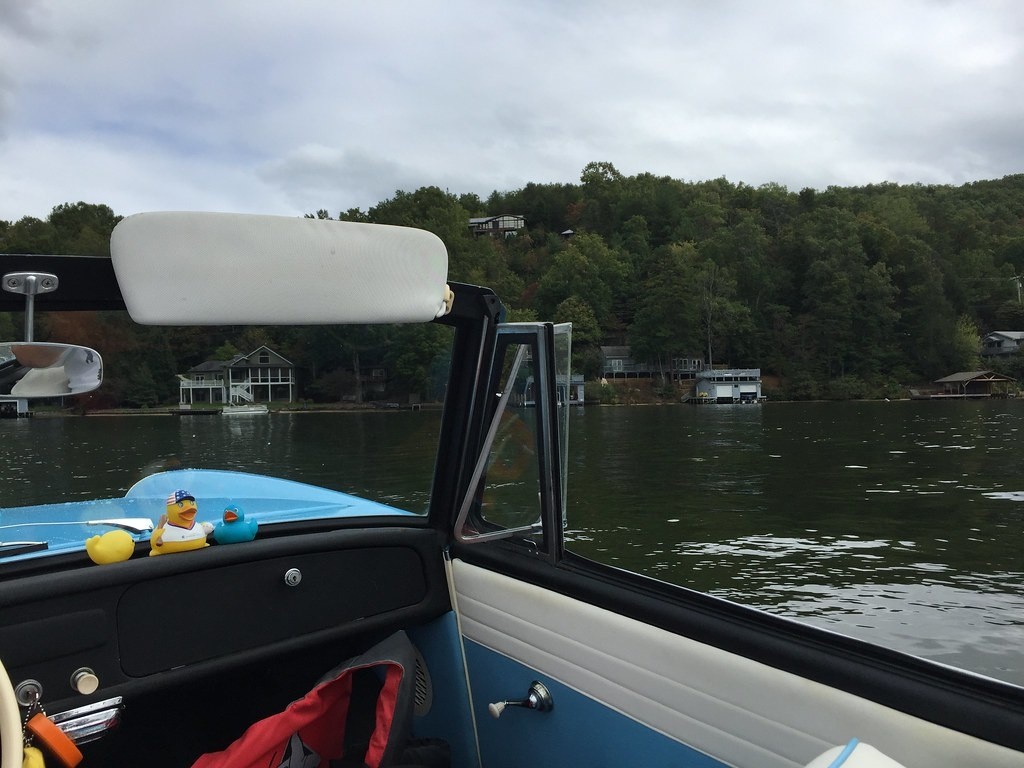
11;344;101;393
